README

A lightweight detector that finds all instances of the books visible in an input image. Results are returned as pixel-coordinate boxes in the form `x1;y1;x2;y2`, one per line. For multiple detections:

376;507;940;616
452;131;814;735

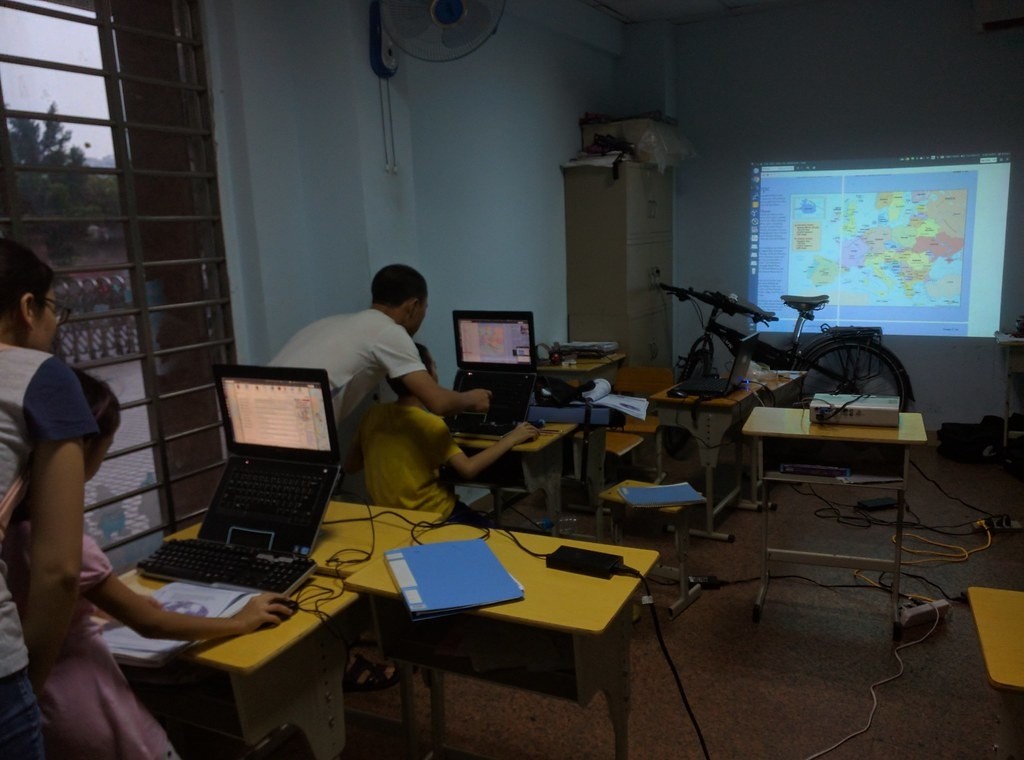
618;481;706;508
781;458;856;477
583;377;649;419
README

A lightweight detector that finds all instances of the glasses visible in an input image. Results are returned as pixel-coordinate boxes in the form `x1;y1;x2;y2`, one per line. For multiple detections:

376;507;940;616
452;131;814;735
36;294;71;326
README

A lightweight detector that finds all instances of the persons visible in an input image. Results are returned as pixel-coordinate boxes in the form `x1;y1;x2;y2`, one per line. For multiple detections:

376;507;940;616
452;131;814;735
348;345;539;523
266;264;491;693
0;237;98;760
2;370;292;760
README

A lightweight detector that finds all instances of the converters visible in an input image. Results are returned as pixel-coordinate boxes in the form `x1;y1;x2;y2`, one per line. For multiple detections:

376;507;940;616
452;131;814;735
857;496;897;511
546;545;623;580
688;575;721;591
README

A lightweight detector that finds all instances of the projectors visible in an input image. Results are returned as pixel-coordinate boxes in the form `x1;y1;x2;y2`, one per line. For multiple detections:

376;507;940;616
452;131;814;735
810;393;900;428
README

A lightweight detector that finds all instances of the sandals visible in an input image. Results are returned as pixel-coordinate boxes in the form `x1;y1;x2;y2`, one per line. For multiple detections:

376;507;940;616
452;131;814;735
341;653;401;693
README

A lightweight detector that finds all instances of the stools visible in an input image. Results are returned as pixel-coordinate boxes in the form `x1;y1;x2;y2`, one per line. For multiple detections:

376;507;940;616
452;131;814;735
596;478;705;621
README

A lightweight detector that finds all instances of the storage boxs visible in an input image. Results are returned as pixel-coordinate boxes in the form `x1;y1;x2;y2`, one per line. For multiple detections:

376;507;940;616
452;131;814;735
581;120;626;151
612;118;681;161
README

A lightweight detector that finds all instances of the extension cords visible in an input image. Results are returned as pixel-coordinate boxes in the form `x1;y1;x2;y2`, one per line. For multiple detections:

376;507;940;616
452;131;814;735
973;519;1022;532
901;599;950;629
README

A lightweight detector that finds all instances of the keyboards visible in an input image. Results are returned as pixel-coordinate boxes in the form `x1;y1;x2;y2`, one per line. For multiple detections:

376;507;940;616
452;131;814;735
445;423;516;440
137;538;318;598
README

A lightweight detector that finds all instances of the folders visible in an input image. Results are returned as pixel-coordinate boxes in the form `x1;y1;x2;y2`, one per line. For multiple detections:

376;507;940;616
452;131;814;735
617;482;708;507
383;539;525;621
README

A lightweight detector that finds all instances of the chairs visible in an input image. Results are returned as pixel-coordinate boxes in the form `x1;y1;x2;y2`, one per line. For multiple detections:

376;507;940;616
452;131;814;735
609;364;676;483
566;377;644;490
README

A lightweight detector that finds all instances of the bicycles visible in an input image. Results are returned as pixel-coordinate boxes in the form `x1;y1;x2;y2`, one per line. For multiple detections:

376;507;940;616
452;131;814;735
656;280;916;414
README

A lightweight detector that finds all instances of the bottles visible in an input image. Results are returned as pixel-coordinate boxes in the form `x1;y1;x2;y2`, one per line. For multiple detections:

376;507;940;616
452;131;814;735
1016;315;1024;334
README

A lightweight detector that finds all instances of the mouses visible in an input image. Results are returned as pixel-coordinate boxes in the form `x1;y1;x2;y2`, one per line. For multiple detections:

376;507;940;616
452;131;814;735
667;389;689;399
259;599;299;629
524;437;534;444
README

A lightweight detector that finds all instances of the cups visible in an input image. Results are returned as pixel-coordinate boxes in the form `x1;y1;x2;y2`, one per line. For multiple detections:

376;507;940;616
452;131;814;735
550;351;561;365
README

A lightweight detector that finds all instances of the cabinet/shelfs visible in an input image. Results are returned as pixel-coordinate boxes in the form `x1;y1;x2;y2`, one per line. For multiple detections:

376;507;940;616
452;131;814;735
568;159;675;367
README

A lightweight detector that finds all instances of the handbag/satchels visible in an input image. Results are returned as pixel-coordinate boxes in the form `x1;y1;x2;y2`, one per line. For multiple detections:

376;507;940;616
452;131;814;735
534;376;596;408
937;415;1005;463
1002;448;1024;481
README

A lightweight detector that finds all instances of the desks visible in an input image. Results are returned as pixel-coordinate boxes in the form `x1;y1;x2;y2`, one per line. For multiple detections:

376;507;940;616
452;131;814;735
537;347;635;378
737;367;813;510
743;402;930;627
652;372;762;540
994;332;1024;447
966;585;1024;760
68;573;363;760
444;416;579;536
160;499;444;760
344;524;668;760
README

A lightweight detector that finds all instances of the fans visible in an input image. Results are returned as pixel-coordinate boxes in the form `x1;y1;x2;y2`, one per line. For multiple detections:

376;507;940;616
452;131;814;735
369;0;508;77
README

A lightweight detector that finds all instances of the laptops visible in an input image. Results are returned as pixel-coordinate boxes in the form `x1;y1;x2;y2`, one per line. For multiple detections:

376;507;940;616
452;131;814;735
452;310;538;425
198;364;342;556
671;332;761;397
